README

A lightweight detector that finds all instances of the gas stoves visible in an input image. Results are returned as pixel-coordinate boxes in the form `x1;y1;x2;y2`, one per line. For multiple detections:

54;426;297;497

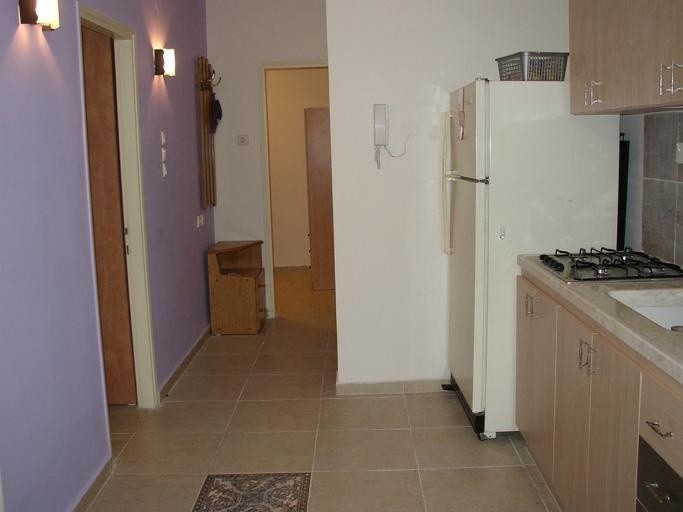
524;244;682;285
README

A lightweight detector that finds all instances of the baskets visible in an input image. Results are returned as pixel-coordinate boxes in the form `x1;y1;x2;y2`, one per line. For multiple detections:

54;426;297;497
495;51;569;81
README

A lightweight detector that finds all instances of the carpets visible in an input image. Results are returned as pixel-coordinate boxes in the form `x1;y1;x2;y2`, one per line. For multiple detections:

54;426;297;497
191;474;309;512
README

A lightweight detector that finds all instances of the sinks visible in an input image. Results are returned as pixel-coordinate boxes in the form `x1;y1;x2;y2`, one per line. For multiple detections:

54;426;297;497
606;286;683;332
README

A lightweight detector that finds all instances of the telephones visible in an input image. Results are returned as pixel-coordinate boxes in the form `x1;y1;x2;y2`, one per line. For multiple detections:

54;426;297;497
374;105;386;146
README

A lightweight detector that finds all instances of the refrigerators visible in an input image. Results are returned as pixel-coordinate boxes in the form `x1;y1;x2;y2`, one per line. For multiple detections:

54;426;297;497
445;79;622;440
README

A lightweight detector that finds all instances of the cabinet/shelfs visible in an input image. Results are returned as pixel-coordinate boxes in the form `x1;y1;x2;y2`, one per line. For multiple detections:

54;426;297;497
205;241;266;336
565;1;683;113
